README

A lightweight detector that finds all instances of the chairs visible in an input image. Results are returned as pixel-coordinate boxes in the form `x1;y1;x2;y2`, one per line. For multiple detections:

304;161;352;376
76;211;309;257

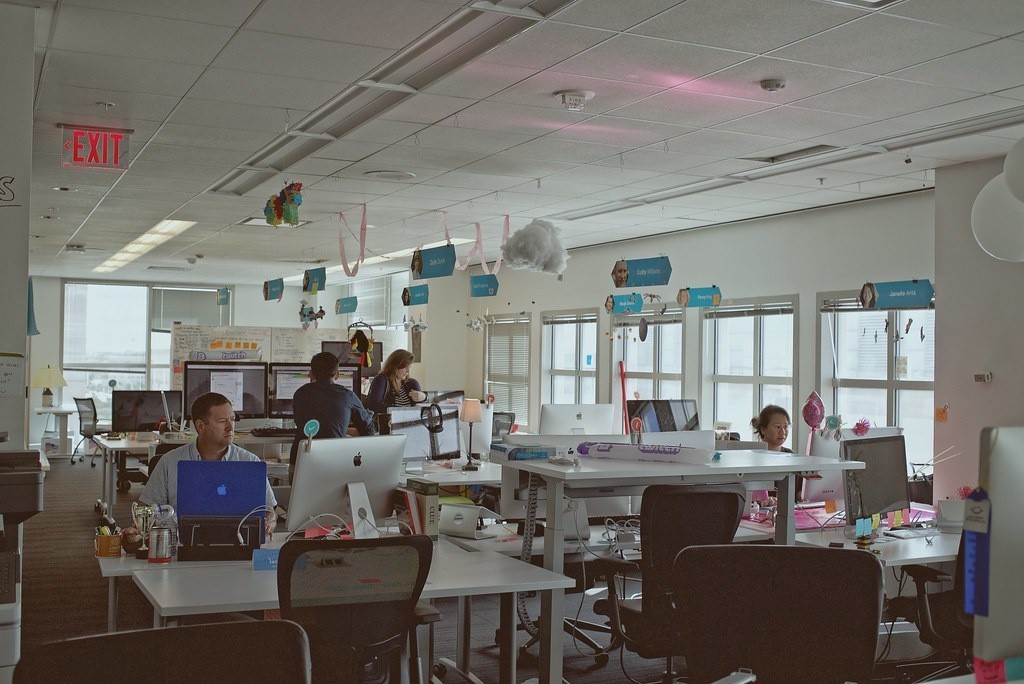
492;518;626;667
13;619;313;684
475;413;517;507
222;537;443;684
673;545;885;684
885;527;974;683
70;399;113;467
593;483;747;684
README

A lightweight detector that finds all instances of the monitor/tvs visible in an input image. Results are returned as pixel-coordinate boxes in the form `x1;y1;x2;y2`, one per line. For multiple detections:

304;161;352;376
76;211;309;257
626;399;701;436
184;361;268;419
540;404;614;435
800;426;901;520
840;435;911;537
269;362;361;419
436;404;494;454
286;432;406;539
387;406;461;462
112;390;182;440
972;425;1024;662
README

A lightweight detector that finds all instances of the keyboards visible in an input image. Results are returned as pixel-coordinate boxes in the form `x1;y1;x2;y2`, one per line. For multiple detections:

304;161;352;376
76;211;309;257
252;427;296;437
883;528;941;539
792;501;826;509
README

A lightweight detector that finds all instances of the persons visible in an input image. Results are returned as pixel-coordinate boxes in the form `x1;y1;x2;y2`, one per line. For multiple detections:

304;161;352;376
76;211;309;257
288;352;375;487
264;282;269;301
613;261;627;287
303;271;310;291
412;252;421;278
678;289;689;306
370;349;429;432
750;404;804;504
860;283;876;308
403;290;411;305
137;391;278;542
604;296;614;313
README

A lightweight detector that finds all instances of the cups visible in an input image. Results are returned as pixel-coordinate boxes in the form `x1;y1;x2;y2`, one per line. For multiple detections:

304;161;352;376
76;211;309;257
94;534;122;559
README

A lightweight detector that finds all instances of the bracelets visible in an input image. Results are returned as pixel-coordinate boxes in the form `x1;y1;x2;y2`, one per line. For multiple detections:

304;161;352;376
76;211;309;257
423;395;427;401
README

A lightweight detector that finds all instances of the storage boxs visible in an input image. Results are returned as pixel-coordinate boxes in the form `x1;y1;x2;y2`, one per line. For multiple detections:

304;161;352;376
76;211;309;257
41;438;72;454
83;425;112;455
490;443;569;461
253;532;306;570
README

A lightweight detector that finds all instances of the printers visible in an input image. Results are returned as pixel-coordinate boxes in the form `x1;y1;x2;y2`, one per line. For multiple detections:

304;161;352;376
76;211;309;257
0;449;50;684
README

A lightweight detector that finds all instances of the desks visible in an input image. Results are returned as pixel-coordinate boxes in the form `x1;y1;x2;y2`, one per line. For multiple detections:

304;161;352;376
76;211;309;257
35;403;962;684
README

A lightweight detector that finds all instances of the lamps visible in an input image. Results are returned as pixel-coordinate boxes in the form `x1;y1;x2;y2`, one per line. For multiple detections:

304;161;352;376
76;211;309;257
32;364;67;407
460;399;483;471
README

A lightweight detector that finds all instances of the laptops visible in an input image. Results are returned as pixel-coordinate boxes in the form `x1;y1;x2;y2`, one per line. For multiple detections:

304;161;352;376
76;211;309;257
178;460;267;544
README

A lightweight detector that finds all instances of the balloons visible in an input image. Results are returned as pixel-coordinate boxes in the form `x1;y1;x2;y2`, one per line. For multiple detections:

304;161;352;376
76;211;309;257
802;390;825;429
971;137;1024;263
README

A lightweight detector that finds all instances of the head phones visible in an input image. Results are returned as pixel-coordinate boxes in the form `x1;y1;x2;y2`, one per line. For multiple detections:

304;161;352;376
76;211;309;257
429;404;444;432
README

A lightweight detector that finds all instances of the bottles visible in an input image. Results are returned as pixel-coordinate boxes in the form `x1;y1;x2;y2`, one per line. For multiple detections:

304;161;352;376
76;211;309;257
750;500;760;520
